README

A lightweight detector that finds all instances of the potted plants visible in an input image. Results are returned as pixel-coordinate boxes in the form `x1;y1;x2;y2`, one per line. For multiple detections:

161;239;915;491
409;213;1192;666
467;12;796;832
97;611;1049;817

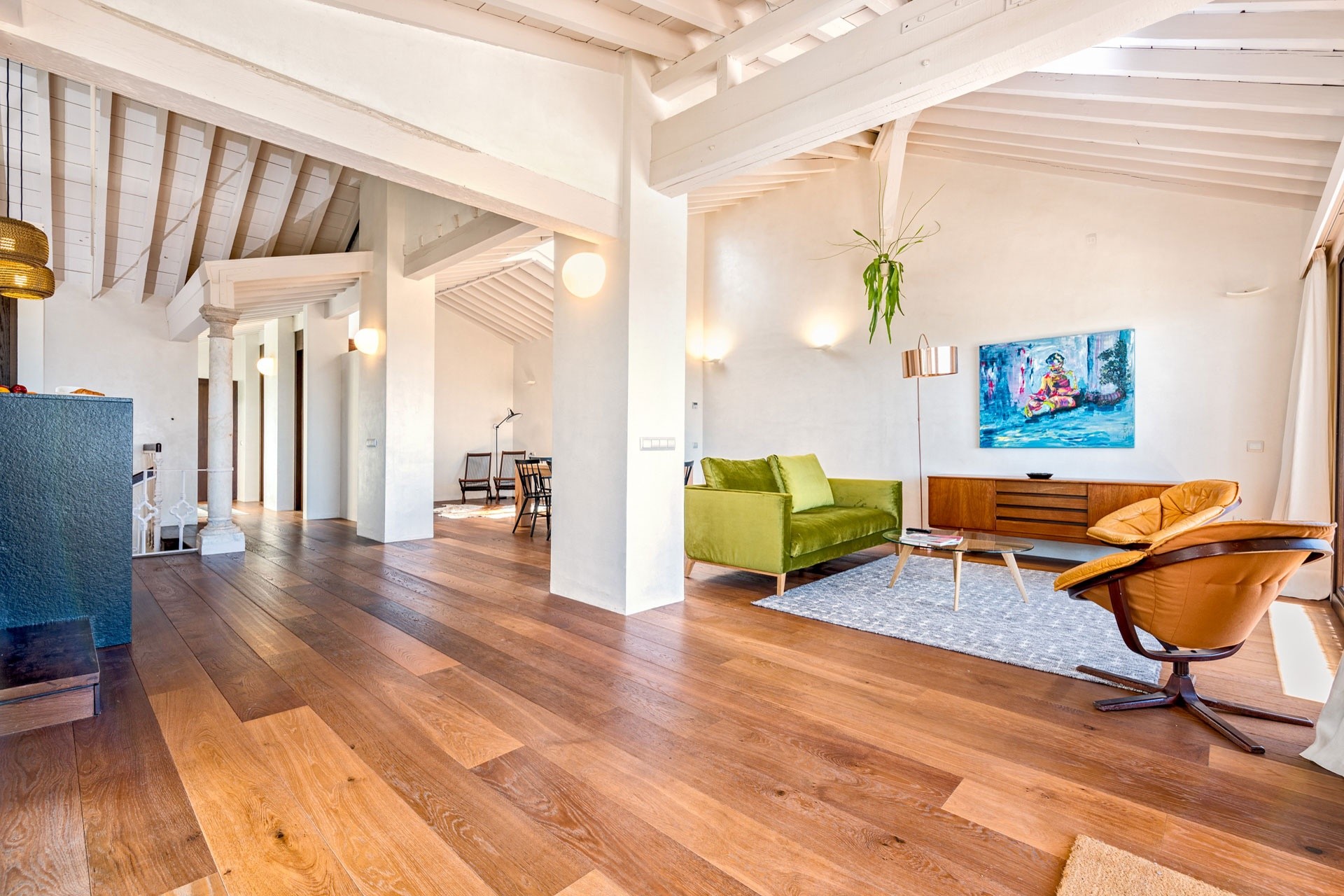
806;157;948;347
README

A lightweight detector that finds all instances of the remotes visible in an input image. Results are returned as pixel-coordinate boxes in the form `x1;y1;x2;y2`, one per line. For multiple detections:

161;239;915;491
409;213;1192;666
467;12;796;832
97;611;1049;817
906;527;931;533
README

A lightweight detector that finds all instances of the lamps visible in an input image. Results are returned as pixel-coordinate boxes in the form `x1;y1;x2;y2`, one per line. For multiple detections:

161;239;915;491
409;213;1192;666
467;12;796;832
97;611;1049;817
352;325;382;357
0;55;57;305
254;355;276;377
487;405;524;501
558;248;611;301
898;332;959;530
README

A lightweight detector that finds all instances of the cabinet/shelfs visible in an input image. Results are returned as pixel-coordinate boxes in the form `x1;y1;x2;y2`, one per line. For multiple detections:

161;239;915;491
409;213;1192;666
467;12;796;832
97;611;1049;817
924;469;1179;546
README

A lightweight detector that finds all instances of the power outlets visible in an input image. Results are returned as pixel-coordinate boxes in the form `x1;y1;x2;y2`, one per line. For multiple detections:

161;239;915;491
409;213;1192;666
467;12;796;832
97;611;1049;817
527;450;536;458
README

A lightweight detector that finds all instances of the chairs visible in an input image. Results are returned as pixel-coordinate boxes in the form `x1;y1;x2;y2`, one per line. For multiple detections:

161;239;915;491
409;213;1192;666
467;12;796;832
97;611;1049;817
458;451;492;505
493;448;552;543
1054;520;1338;758
1082;476;1246;551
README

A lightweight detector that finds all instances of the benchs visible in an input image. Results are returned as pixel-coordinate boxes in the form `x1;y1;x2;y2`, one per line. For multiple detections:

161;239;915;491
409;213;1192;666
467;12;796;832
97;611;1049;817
683;452;904;595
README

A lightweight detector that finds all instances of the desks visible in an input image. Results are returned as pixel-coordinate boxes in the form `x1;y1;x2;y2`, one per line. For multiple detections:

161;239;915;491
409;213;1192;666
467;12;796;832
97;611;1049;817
514;463;551;527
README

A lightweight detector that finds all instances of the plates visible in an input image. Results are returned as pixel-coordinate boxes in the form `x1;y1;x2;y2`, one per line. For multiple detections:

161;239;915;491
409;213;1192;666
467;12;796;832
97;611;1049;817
1026;473;1053;479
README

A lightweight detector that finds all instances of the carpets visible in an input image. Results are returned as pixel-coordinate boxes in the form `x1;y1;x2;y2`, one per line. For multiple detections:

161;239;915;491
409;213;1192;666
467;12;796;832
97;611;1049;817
1266;600;1344;705
433;502;553;520
748;548;1167;696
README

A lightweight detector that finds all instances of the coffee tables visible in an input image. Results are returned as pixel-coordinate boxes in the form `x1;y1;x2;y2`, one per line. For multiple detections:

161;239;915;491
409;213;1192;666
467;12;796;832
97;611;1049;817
876;523;1039;614
